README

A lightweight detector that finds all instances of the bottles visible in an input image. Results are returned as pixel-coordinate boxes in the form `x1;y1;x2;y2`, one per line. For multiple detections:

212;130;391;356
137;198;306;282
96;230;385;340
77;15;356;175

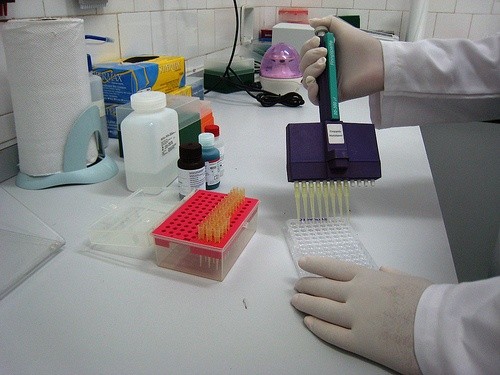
204;125;224;177
88;54;108;148
120;91;179;194
177;143;206;199
199;133;220;189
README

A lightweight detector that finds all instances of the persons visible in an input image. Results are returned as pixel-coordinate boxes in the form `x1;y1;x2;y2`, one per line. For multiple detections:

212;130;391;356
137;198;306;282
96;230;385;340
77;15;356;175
290;16;500;375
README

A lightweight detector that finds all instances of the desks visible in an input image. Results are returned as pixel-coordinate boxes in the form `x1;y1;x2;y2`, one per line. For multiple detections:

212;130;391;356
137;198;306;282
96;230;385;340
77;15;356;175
0;75;459;375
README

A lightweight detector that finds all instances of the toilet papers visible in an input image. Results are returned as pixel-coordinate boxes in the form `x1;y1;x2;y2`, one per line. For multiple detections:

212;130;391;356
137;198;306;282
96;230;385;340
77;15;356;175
2;17;98;179
